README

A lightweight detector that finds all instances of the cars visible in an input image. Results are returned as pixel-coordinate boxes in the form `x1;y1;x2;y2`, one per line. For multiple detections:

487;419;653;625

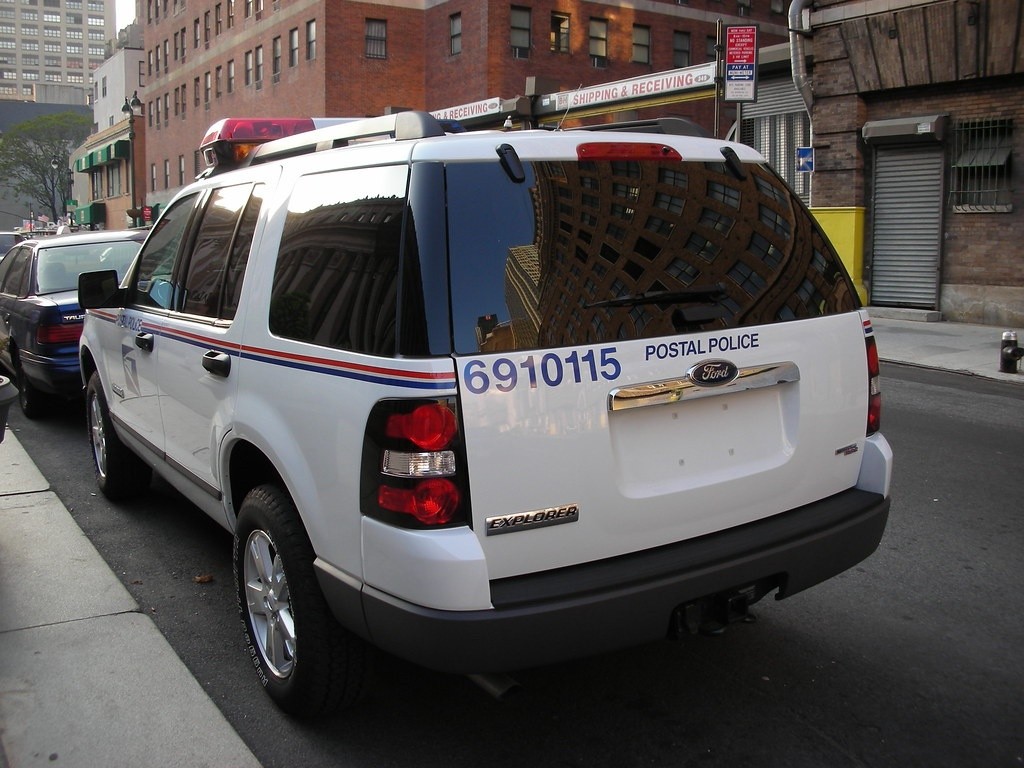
0;232;25;262
0;229;156;418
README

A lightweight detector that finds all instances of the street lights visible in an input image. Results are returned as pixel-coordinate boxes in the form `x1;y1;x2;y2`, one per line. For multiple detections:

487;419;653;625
121;90;143;229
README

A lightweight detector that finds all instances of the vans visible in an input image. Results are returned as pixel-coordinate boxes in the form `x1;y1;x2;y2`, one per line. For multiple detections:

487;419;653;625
78;108;896;721
